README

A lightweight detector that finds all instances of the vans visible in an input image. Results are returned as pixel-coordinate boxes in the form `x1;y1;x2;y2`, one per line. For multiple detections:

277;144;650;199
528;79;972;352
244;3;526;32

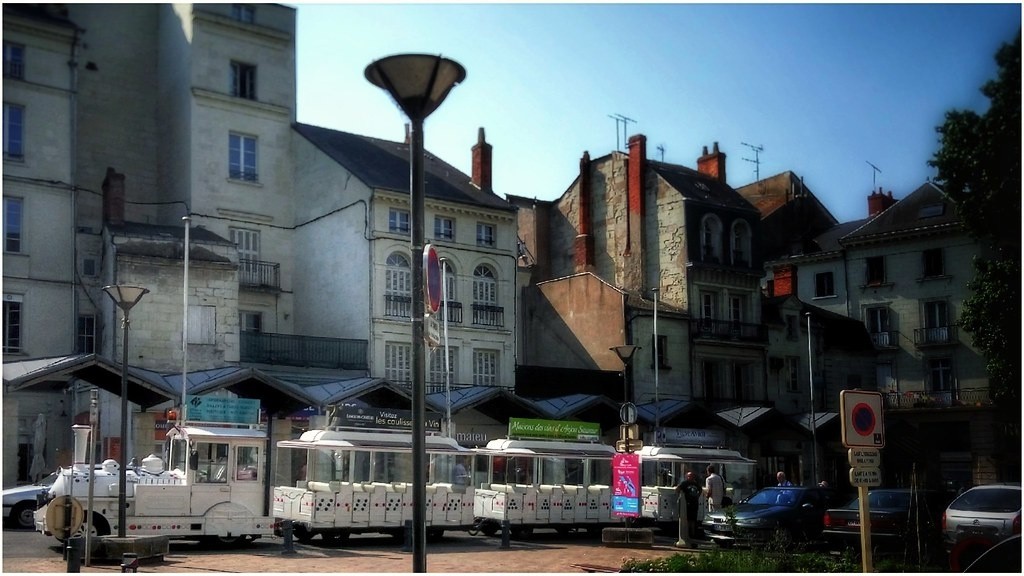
941;481;1021;552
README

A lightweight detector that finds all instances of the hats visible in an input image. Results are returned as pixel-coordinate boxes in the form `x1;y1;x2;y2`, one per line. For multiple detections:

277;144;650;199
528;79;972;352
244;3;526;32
818;481;829;488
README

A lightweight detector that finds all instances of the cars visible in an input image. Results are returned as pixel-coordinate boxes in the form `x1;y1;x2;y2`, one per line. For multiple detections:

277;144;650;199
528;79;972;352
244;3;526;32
1;462;161;530
822;489;940;547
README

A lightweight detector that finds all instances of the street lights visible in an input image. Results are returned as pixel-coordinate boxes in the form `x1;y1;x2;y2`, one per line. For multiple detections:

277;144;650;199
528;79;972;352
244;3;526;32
610;344;643;527
364;50;468;573
101;284;151;539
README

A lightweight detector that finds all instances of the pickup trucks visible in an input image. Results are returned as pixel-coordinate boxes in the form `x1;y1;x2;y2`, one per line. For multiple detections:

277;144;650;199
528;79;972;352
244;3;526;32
702;486;851;555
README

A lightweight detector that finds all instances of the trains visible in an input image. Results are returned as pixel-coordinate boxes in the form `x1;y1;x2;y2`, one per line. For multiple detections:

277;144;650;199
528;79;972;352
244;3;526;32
34;416;758;542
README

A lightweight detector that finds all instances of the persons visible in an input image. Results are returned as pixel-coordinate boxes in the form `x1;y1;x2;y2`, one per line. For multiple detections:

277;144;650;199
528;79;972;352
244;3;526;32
773;470;829;505
704;465;723;513
452;456;470;485
675;472;703;538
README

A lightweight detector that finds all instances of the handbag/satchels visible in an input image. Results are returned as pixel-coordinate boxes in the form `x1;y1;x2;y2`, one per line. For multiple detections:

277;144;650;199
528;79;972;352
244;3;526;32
721;497;732;508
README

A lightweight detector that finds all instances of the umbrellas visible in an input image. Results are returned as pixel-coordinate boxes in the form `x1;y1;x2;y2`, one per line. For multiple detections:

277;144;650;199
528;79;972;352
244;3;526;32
31;413;47;484
